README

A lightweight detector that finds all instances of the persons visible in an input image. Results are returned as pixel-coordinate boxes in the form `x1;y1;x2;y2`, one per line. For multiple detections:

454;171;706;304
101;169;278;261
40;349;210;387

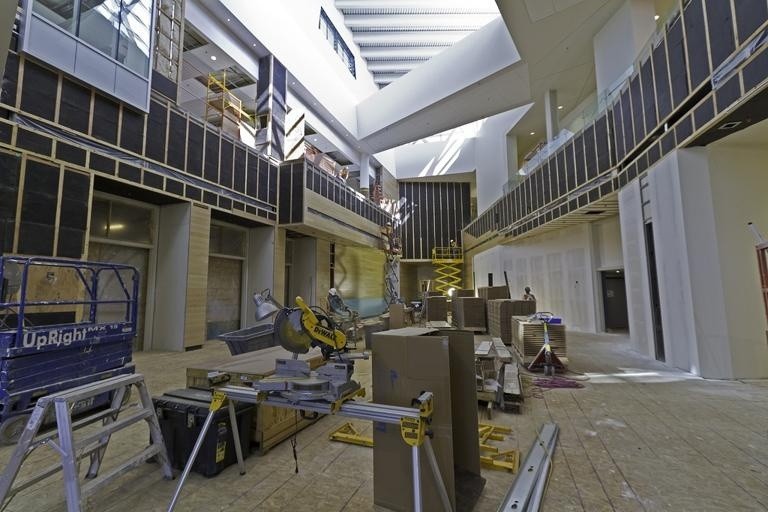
336;166;349;183
385;222;393;249
523;287;535;301
450;240;457;259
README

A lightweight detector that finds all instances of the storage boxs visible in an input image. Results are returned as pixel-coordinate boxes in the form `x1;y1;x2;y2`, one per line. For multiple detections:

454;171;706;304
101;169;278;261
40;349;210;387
150;388;258;479
364;321;384;350
457;297;485;327
371;325;480;510
389;304;405;329
219;323;279;357
426;296;447;322
